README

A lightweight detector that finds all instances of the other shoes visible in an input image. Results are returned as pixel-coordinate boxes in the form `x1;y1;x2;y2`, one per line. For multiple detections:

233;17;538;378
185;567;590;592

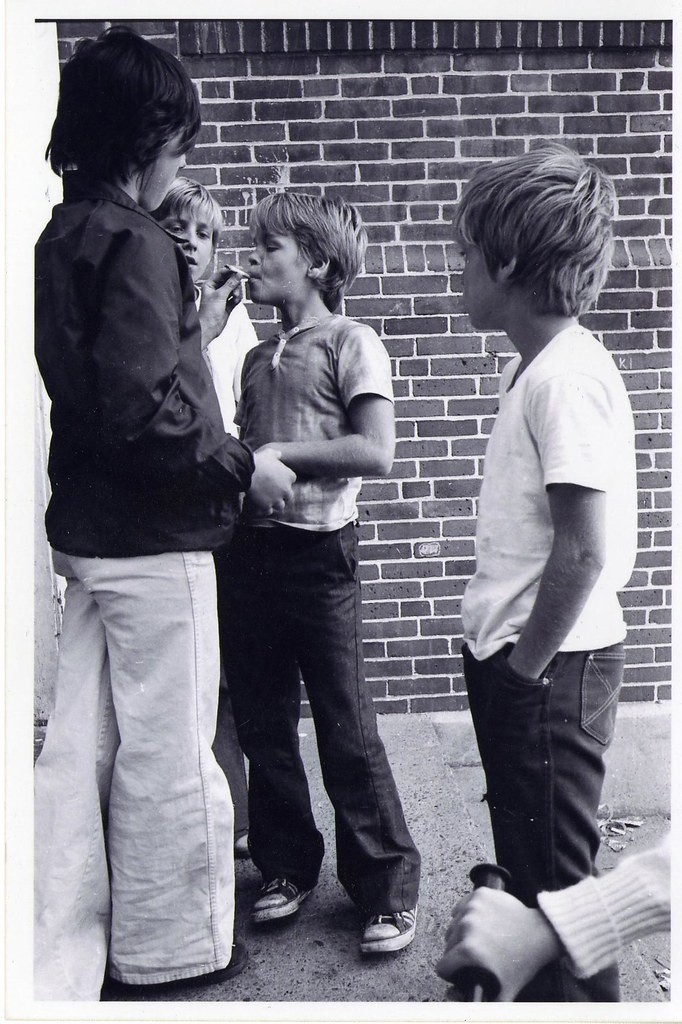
124;939;249;994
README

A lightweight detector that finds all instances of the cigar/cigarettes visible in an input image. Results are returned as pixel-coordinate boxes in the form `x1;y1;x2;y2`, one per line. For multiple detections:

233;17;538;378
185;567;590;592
225;264;252;279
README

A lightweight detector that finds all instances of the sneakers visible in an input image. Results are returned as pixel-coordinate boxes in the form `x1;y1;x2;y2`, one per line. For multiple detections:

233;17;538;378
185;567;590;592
252;880;312;921
360;904;418;952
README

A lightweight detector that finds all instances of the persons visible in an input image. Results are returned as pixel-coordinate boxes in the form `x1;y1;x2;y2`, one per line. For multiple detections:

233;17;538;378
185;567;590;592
436;831;671;1003
233;193;422;953
35;30;298;1001
154;176;261;855
460;142;638;1000
